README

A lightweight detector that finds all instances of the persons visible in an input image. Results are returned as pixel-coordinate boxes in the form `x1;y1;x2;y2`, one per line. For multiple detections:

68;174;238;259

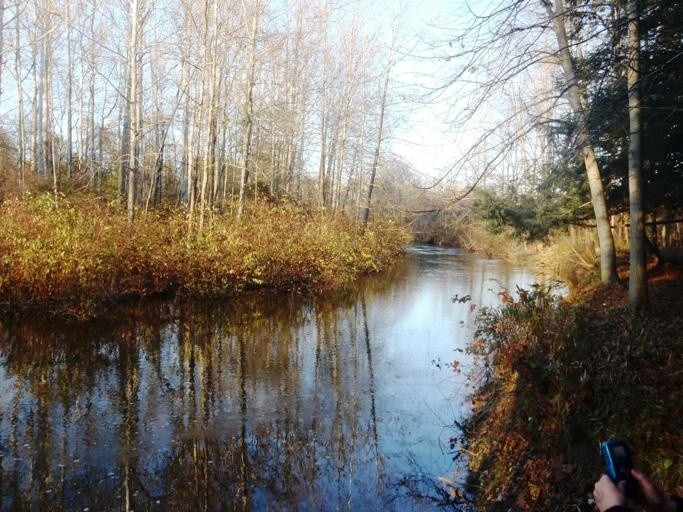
592;468;682;512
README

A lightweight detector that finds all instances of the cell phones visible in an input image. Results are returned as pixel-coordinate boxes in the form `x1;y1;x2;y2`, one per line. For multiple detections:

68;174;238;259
602;439;633;492
